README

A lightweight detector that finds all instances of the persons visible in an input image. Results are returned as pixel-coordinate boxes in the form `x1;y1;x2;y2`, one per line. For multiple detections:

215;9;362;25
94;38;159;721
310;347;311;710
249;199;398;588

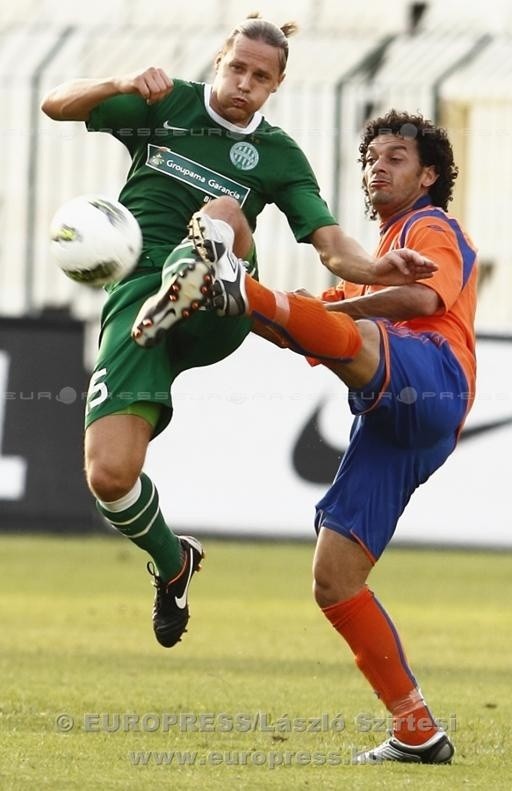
187;109;477;764
40;13;441;648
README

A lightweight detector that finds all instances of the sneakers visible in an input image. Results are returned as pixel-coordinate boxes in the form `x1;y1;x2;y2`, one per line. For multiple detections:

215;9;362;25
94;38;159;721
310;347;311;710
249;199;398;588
130;262;211;348
147;535;205;647
187;211;245;322
357;728;454;762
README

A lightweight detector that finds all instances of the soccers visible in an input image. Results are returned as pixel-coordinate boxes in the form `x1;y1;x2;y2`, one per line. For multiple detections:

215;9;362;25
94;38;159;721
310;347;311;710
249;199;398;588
50;197;144;285
